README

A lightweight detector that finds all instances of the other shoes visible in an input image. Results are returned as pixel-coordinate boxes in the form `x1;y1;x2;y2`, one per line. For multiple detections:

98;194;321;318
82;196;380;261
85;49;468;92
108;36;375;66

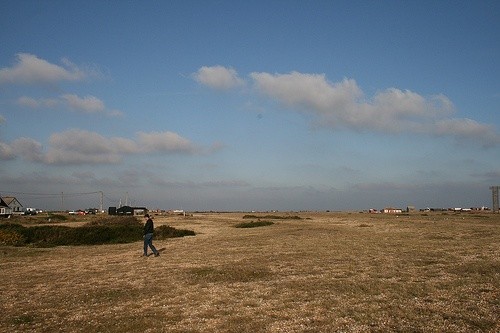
141;254;147;257
154;252;159;257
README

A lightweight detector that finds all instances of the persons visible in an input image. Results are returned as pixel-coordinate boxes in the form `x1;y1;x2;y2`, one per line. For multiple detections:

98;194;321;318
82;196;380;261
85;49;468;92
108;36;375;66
142;214;159;258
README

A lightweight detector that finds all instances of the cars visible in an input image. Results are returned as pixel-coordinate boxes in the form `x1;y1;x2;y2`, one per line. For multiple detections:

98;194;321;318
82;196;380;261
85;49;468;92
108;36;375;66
22;208;99;218
